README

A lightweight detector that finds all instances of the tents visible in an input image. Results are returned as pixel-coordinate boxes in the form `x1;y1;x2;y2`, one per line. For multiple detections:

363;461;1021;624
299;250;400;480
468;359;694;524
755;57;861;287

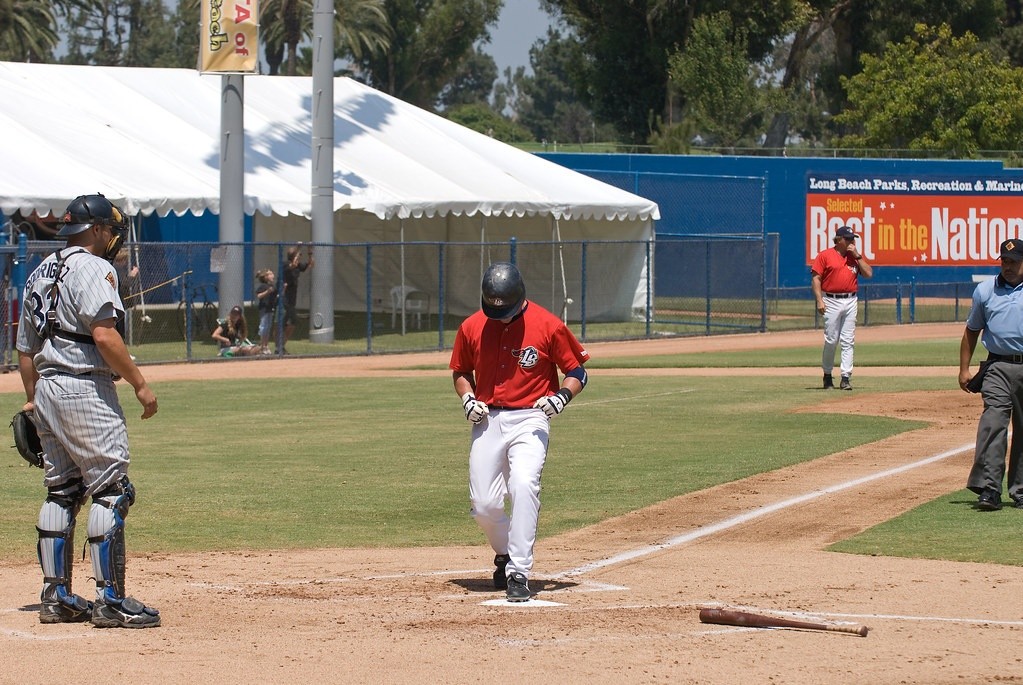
0;59;661;323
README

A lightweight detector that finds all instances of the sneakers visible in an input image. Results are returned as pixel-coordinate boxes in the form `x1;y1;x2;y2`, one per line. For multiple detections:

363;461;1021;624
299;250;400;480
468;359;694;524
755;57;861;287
977;490;1002;510
823;373;835;388
493;554;511;590
40;602;92;624
840;377;852;390
506;572;530;602
1014;497;1023;509
92;601;161;628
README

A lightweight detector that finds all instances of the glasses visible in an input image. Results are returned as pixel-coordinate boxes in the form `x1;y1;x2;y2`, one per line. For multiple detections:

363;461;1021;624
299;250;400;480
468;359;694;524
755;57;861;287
843;237;855;241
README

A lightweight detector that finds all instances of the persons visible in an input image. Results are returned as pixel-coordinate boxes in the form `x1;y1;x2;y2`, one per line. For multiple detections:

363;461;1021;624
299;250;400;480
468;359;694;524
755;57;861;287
274;244;314;354
112;249;139;361
450;262;590;603
211;307;264;355
16;192;161;629
811;226;873;388
257;269;288;354
959;239;1023;510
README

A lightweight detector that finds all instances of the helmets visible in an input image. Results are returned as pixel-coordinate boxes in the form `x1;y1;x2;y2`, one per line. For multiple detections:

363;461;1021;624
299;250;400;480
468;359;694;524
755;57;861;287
481;262;525;319
56;195;112;236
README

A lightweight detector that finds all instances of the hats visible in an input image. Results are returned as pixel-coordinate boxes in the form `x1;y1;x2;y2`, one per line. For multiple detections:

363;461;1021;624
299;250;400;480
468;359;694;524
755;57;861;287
997;239;1023;261
836;226;859;238
230;306;243;315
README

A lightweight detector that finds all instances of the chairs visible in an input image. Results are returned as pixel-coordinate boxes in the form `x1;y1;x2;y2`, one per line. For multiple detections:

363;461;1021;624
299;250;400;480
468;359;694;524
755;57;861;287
391;284;431;329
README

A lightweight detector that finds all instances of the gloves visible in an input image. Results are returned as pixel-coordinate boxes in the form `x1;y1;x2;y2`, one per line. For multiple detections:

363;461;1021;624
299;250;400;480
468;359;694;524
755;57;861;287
532;391;567;418
462;395;489;425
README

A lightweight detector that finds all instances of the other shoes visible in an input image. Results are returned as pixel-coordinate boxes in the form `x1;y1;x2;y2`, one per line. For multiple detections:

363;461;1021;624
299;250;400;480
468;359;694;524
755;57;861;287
275;348;289;355
259;346;271;355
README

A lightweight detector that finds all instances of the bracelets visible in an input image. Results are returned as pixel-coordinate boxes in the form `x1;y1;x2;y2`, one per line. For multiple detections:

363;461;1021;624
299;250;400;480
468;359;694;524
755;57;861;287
856;254;862;259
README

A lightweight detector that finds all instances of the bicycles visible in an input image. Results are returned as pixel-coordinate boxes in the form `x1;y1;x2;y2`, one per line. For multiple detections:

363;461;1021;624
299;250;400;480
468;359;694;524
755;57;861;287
176;282;218;342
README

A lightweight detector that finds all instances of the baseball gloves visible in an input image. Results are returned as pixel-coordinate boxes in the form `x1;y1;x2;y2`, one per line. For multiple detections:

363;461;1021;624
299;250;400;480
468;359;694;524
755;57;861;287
12;412;45;468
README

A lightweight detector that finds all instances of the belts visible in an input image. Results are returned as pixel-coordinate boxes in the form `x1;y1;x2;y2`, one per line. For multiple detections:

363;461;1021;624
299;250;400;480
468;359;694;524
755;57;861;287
988;352;1023;364
487;404;533;409
826;293;855;298
58;371;92;375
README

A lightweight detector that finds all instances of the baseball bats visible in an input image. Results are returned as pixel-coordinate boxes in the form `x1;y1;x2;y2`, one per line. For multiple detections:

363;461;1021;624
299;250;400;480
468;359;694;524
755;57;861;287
699;608;868;637
